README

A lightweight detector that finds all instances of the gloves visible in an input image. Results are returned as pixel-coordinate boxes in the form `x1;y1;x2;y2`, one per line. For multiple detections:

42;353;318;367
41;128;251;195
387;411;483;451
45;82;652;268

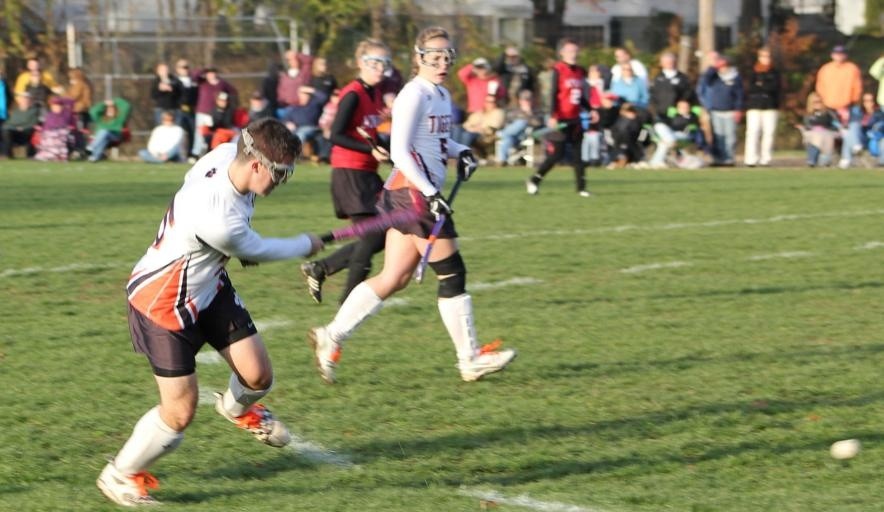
424;193;452;220
456;148;479;183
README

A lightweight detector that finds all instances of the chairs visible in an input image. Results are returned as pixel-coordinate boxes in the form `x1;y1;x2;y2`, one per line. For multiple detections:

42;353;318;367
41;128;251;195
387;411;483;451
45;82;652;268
795;100;884;169
26;112;131;163
493;101;711;171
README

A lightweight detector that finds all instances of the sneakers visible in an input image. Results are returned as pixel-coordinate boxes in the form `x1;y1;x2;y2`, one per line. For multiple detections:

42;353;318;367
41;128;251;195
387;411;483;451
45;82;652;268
456;343;519;384
300;257;325;305
309;323;341;386
211;392;293;448
96;459;168;509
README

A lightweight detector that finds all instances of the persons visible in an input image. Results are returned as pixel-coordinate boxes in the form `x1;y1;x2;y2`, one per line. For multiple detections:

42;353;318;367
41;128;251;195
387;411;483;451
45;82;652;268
300;37;393;307
525;38;593;199
307;28;517;385
92;117;326;506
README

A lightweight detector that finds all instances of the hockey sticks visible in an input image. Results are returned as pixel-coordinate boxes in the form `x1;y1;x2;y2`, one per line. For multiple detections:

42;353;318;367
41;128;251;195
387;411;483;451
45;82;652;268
531;117;592;155
415;179;461;285
238;188;428;268
357;127;386;155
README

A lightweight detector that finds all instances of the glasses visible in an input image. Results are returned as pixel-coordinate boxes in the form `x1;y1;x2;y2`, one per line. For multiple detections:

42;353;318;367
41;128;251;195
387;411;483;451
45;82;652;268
268;160;296;187
416;43;457;67
360;52;395;79
